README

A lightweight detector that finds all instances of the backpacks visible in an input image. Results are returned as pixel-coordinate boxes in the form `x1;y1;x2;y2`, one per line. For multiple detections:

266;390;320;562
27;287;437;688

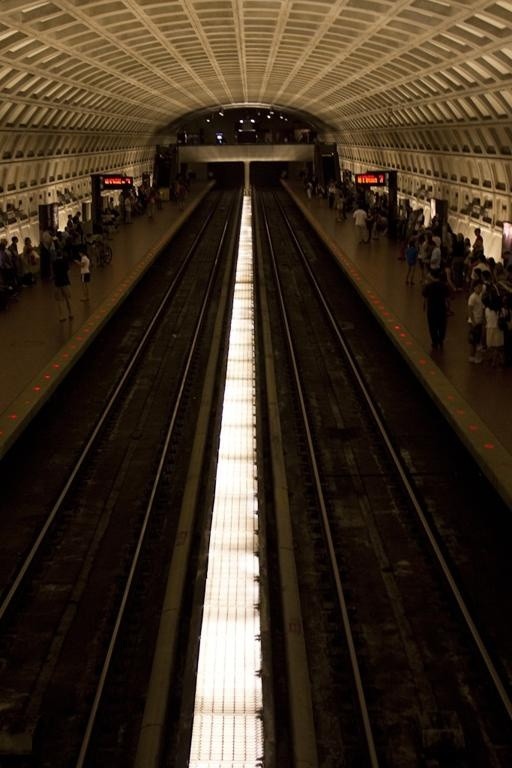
485;282;503;309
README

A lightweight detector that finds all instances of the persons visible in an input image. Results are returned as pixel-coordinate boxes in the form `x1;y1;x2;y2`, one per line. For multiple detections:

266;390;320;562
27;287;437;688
301;170;364;222
405;227;512;374
1;169;196;304
49;245;75;322
353;191;425;262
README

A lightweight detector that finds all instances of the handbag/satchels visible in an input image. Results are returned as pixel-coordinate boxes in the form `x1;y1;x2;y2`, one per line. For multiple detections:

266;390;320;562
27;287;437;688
497;312;507;332
467;323;483;345
